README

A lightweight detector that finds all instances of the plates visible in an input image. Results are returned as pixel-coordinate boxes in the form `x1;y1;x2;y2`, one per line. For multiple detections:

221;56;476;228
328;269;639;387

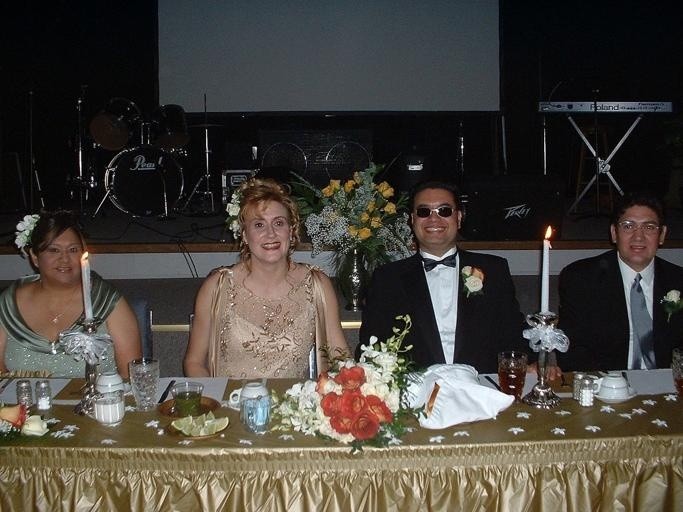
168;420;230;440
160;397;219;417
595;394;638;404
231;403;278;412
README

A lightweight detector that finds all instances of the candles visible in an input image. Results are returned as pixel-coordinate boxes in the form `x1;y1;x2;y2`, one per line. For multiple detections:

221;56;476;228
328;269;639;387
81;251;94;318
540;225;552;312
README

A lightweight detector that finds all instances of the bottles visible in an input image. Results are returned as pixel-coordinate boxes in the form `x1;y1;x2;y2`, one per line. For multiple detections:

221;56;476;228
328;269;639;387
573;372;585;400
35;379;54;415
579;375;595;407
15;379;34;410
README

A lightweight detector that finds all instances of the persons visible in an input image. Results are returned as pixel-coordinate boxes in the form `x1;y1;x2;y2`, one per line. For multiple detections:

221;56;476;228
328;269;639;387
183;178;352;378
354;183;562;383
0;211;143;380
557;190;682;371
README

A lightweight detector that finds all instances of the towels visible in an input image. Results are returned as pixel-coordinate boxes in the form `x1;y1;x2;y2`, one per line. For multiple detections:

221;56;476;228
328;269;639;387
401;358;516;429
157;377;229;402
0;376;72;406
608;368;679;395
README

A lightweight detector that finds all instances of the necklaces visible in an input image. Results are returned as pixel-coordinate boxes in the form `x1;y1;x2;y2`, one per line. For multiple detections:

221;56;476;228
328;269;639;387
36;281;81;323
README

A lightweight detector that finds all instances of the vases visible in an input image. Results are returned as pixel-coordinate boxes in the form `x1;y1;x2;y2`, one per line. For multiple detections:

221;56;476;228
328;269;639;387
337;248;369;312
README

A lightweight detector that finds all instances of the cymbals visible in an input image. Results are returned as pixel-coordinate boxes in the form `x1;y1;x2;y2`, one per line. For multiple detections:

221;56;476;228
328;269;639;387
192;124;223;128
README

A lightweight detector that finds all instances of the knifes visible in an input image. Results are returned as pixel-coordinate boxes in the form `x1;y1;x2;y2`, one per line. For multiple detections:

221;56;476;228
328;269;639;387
0;377;14;393
158;380;177;404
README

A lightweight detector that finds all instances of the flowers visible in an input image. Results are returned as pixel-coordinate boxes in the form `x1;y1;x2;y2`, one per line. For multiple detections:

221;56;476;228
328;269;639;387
656;289;683;326
304;162;415;279
277;314;424;446
459;265;486;299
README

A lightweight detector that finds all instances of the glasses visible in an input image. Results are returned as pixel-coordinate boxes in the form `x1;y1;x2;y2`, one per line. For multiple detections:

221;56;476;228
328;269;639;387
618;221;659;232
413;205;456;218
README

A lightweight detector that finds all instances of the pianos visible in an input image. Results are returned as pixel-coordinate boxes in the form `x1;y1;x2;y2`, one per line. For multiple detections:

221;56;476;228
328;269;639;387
538;102;672;113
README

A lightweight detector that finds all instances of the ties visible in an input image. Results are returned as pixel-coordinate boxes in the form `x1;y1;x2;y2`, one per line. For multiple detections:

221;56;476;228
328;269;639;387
630;273;656;370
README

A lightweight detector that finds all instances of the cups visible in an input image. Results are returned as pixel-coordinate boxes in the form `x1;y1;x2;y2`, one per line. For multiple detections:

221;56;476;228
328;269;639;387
241;399;272;433
93;370;126;428
229;381;270;409
670;347;683;399
594;373;630;398
171;382;205;417
496;350;529;400
128;358;161;412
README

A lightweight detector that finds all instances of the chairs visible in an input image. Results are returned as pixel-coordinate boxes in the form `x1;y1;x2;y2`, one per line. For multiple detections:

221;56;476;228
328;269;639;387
131;298;154;361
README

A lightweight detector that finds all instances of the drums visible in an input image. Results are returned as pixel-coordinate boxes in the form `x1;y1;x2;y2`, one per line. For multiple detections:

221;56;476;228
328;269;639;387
104;145;184;218
91;97;144;151
261;142;307;186
150;104;190;153
326;141;370;187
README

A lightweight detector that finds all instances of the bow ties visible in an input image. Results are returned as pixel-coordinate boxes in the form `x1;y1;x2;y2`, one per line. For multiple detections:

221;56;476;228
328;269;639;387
418;251;457;272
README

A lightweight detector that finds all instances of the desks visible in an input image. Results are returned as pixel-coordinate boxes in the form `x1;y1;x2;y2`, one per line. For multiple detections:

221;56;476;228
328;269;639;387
538;100;673;216
